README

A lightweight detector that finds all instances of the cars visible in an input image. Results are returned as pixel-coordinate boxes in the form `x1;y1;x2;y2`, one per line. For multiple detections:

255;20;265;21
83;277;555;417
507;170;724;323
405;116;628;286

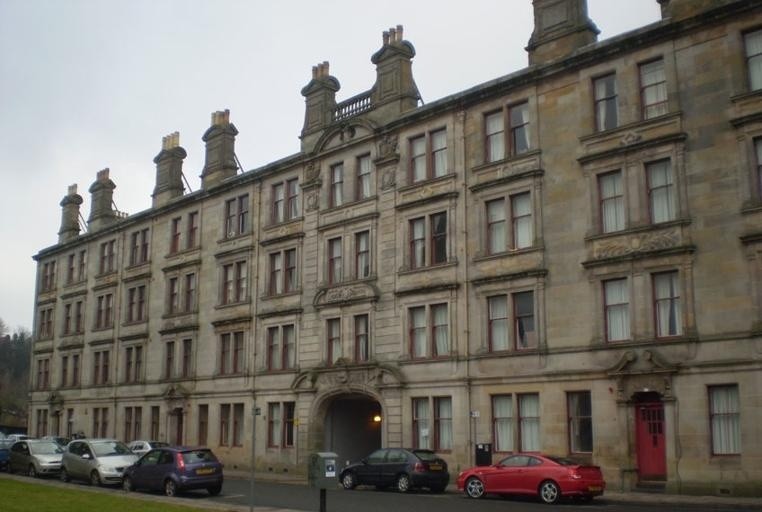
456;450;605;502
0;433;168;489
121;446;224;499
338;447;449;495
